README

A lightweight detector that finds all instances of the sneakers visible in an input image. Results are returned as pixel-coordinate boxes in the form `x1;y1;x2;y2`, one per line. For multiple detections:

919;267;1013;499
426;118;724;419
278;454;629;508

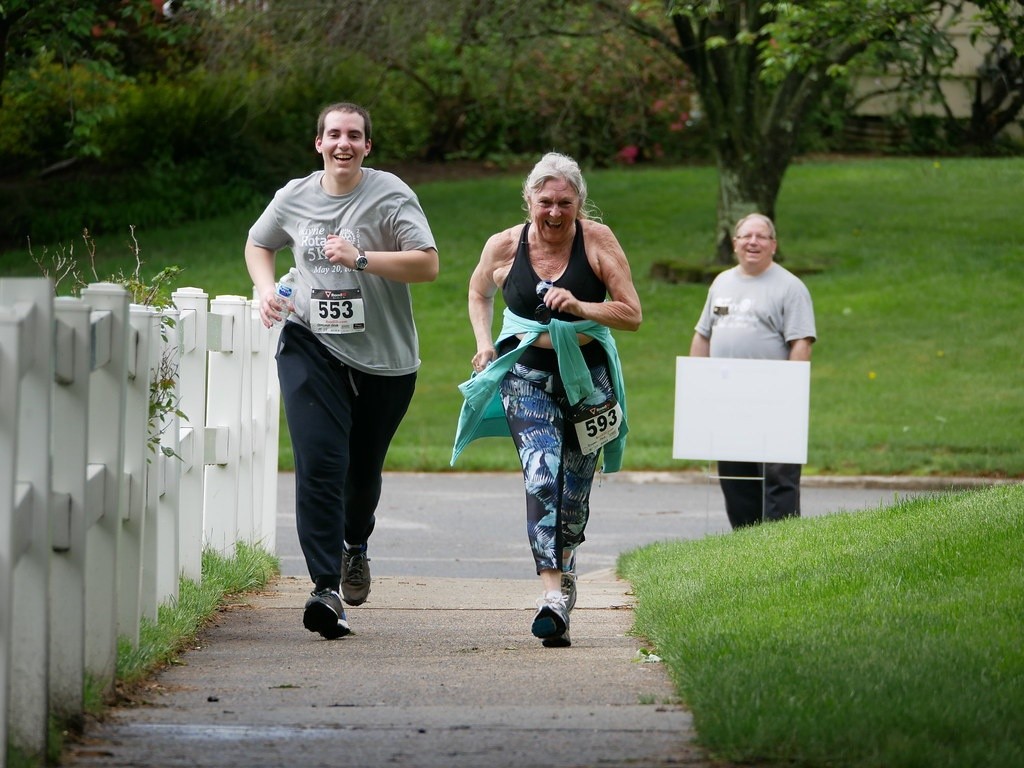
530;591;571;647
303;588;350;640
340;541;371;606
561;549;577;614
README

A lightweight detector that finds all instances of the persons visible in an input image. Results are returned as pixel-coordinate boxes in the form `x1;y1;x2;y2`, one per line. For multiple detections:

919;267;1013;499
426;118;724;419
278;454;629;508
468;151;641;648
242;102;439;640
689;212;816;533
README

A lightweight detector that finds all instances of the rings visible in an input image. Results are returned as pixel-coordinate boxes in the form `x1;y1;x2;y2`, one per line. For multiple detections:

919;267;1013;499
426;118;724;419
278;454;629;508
476;366;480;370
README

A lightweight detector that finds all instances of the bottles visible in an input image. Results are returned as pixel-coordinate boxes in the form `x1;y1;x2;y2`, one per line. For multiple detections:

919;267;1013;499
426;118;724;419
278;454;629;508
261;267;300;330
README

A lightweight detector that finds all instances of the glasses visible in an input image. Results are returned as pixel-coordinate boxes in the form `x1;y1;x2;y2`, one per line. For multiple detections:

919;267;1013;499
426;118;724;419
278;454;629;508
736;233;774;242
535;279;553;325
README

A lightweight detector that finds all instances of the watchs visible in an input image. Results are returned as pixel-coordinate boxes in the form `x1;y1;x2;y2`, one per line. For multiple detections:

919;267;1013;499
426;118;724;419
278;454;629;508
355;248;368;270
